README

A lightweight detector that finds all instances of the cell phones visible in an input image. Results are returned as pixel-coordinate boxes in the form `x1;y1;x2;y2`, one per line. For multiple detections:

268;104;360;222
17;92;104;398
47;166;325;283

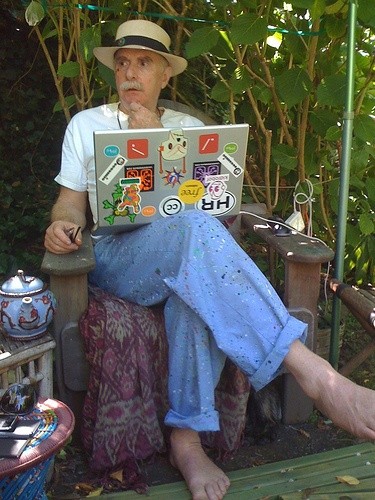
266;216;290;237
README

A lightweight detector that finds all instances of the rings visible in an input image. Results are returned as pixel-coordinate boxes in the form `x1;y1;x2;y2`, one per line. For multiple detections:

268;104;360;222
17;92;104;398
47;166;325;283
128;118;131;122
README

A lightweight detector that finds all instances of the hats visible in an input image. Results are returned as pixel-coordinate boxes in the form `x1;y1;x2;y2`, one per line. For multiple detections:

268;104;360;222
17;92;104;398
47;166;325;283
93;20;187;77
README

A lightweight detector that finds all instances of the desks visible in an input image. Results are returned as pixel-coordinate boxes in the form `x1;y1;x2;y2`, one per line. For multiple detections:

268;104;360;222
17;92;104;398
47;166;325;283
0;395;75;500
0;334;57;397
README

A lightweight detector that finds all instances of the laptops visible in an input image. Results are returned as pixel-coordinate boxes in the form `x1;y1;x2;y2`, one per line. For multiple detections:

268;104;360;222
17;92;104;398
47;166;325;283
90;124;250;236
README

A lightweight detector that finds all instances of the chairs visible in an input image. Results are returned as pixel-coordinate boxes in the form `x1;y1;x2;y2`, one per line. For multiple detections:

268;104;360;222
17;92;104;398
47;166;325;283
40;101;334;471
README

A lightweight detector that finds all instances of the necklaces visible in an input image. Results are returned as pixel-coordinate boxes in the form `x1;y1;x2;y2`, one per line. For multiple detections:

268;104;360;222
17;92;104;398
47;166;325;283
117;104;161;130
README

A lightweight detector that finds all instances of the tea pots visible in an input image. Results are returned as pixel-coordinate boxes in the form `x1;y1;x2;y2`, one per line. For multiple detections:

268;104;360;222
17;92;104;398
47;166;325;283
0;270;57;340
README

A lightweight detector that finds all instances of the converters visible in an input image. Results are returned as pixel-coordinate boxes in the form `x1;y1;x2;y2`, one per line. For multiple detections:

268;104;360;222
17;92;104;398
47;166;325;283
285;211;306;232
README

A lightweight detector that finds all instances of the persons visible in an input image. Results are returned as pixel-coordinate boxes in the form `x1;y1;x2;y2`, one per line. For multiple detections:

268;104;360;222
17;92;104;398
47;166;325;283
44;20;375;500
